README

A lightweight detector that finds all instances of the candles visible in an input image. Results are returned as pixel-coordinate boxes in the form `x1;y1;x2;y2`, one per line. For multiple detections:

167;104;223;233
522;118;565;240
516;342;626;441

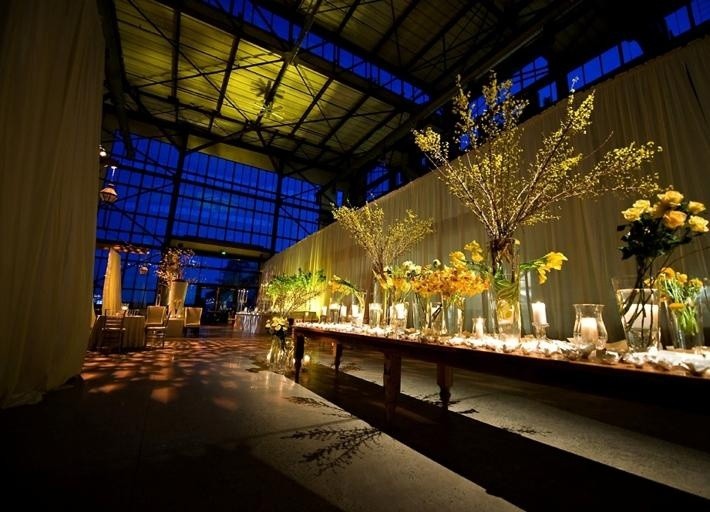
574;302;607;350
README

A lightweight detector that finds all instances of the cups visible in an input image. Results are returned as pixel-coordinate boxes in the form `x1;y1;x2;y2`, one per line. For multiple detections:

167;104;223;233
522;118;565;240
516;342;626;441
330;303;340;323
390;302;408;328
448;297;466;335
573;274;704;351
369;303;381;327
351;305;364;328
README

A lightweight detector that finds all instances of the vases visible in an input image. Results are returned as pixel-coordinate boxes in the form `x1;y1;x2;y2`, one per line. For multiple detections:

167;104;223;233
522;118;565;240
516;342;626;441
614;281;703;350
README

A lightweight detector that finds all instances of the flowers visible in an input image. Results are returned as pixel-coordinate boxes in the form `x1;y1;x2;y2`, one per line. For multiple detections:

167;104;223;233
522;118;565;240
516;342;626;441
412;63;665;269
329;197;436;271
615;185;710;263
263;269;367;311
644;265;708;323
371;241;569;321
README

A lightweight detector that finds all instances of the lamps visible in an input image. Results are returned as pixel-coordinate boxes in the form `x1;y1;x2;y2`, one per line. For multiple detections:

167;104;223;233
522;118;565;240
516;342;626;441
98;176;120;209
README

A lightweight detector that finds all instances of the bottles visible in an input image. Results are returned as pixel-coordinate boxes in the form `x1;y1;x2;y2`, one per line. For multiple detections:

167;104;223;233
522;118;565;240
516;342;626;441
155;290;162;307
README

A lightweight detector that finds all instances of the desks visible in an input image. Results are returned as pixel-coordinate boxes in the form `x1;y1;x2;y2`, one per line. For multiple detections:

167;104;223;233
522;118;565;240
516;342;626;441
290;325;708;445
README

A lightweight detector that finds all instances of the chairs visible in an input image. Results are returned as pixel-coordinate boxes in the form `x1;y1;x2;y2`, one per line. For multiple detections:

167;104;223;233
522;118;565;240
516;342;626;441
183;306;203;337
90;305;175;354
